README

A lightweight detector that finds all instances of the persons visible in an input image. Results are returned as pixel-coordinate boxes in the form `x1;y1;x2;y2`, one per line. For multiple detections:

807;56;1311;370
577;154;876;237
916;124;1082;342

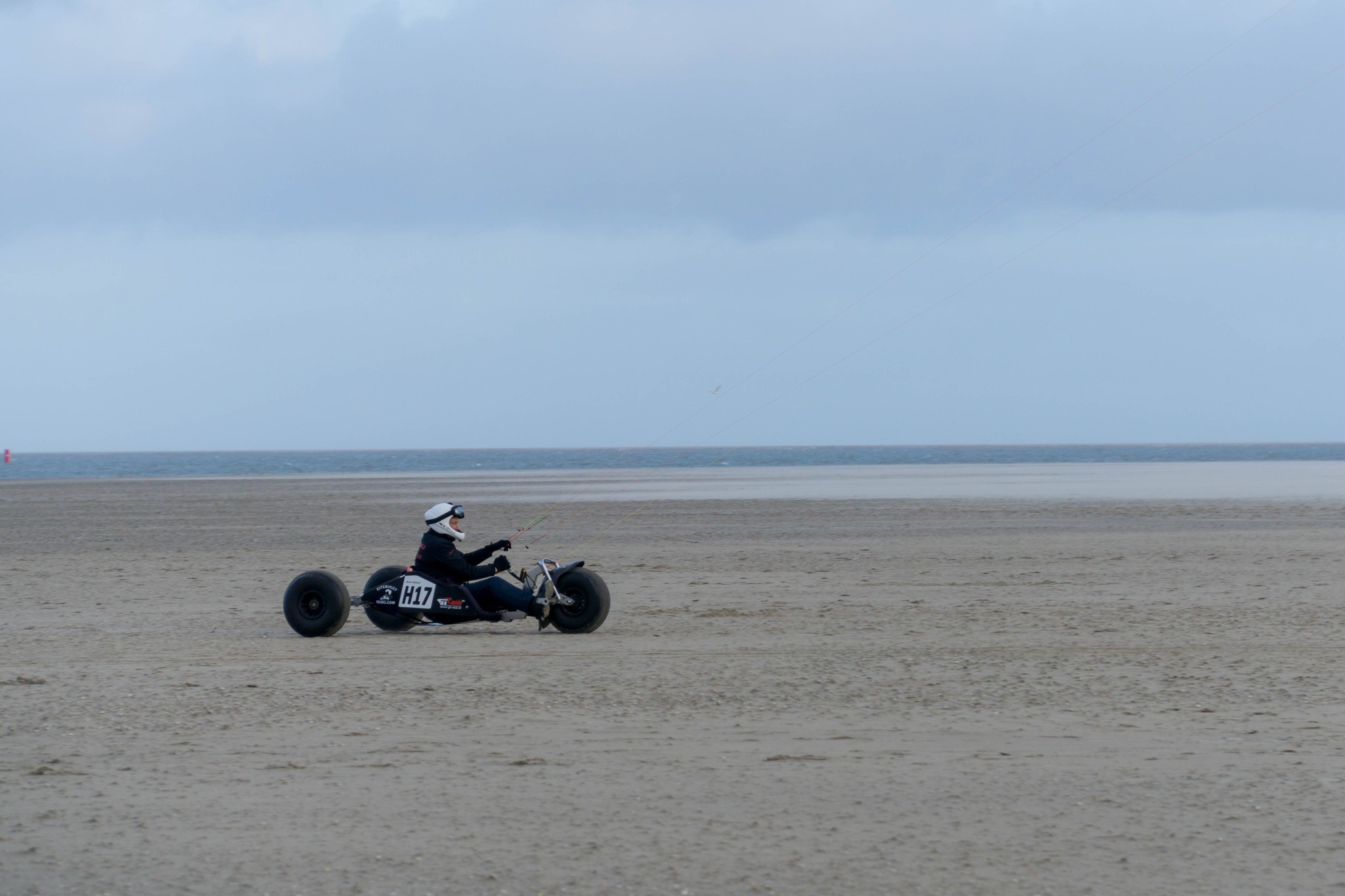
415;502;554;617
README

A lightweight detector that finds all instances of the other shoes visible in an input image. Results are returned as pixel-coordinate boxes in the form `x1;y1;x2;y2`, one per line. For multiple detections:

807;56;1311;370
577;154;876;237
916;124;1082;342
532;580;554;617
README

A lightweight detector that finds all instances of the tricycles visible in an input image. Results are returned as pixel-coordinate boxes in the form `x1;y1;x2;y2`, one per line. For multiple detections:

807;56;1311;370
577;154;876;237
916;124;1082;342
281;557;609;638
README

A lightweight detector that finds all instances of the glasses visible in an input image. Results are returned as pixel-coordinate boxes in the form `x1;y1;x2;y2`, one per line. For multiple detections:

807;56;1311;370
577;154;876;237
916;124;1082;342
451;505;464;519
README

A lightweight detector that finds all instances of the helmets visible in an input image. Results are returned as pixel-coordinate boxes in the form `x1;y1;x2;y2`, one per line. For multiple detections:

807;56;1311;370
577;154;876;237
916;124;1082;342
424;503;465;542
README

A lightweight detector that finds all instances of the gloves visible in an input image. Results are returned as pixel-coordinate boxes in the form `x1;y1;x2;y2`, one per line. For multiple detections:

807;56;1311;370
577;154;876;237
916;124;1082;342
493;555;511;573
487;540;511;551
